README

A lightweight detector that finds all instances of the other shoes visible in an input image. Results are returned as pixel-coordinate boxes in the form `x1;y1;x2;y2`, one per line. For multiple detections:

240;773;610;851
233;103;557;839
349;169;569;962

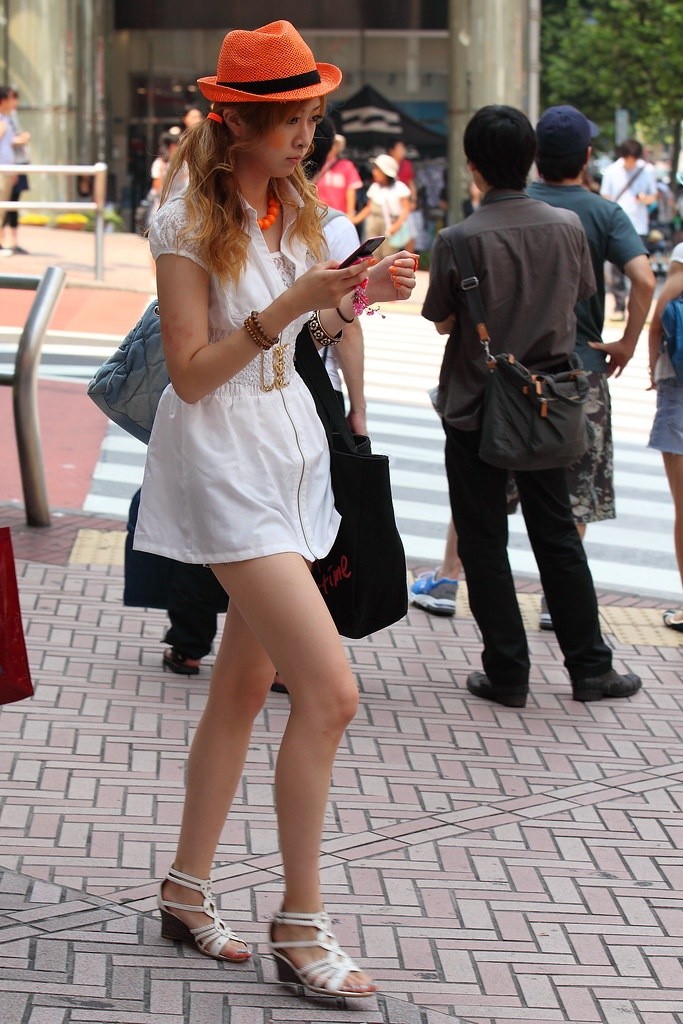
611;308;626;321
663;607;683;631
0;243;30;256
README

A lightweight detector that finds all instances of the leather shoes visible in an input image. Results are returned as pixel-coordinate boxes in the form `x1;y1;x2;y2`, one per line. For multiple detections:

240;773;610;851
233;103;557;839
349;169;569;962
467;670;525;708
569;666;642;704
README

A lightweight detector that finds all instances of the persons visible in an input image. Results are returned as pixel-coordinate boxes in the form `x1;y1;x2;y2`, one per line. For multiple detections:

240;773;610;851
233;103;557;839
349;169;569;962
145;103;205;278
0;85;30;257
353;139;420;267
646;160;683;630
133;20;421;997
316;132;363;225
409;105;658;629
582;139;683;320
122;197;373;691
422;105;644;708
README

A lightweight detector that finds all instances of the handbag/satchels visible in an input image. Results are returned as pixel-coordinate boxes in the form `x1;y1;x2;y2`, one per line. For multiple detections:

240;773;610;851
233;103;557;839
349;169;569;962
87;299;171;445
478;352;589;471
298;324;409;639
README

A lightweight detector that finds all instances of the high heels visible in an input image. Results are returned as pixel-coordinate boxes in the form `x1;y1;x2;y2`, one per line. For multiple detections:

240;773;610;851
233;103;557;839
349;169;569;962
268;902;373;997
158;864;247;963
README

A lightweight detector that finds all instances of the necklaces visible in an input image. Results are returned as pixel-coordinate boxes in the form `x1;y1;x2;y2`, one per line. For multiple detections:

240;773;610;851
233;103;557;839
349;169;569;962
255;190;282;231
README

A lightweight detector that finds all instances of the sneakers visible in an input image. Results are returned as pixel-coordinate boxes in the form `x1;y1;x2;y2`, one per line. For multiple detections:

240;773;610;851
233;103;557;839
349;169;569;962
539;599;556;632
415;566;457;614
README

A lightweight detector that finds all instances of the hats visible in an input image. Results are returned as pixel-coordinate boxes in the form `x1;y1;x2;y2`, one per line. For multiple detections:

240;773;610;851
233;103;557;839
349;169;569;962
371;155;399;179
159;126;183;144
196;18;343;103
536;105;601;157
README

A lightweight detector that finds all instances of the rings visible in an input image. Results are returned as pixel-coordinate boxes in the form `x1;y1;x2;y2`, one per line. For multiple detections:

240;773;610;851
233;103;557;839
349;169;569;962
413;256;418;270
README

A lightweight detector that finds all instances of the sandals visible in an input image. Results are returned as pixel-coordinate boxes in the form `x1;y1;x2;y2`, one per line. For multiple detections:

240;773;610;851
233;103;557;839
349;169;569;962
163;645;200;675
270;672;289;691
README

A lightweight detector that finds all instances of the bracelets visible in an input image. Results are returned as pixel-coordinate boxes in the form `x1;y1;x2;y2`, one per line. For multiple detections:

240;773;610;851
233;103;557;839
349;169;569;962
335;304;357;324
243;310;279;350
307;309;344;347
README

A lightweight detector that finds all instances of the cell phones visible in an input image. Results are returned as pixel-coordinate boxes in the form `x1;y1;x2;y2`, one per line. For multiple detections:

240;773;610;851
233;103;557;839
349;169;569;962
337;235;386;270
